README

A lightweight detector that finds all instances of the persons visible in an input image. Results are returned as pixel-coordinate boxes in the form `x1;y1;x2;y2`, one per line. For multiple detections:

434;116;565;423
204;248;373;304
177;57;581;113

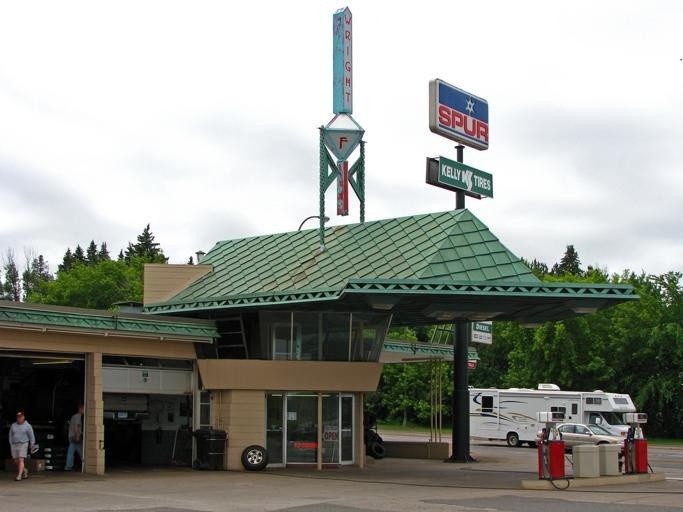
9;411;36;481
64;404;84;470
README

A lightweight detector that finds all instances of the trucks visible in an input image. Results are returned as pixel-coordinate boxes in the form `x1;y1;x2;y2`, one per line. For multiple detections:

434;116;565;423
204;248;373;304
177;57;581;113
468;383;647;451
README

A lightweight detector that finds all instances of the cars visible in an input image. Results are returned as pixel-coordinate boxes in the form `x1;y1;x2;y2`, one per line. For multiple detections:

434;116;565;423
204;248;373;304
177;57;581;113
536;422;628;457
287;430;331;460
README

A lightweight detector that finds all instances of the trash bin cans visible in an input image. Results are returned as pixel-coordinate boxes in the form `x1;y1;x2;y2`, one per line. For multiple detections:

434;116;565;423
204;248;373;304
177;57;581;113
192;429;226;469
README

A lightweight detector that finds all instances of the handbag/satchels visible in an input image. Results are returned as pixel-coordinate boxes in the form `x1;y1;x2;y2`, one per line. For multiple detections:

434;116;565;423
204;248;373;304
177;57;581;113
71;436;82;444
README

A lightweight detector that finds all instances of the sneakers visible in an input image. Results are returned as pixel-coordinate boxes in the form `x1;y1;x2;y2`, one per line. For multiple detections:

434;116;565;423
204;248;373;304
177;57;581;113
16;469;28;480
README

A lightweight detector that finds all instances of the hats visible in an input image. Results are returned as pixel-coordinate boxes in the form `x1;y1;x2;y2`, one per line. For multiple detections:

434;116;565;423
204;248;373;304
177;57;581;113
17;412;24;416
